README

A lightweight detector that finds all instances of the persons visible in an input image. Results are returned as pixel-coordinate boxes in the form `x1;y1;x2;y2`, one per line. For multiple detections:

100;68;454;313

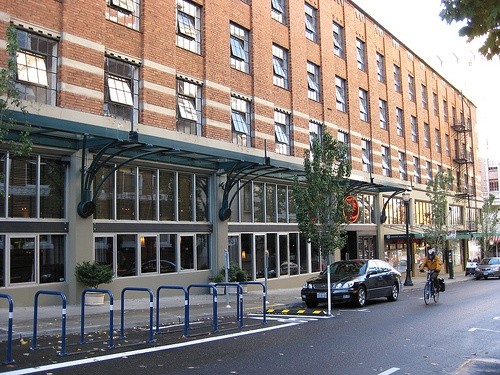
420;249;442;297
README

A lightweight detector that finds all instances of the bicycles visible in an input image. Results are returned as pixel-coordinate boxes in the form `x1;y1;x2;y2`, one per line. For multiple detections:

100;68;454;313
422;268;445;304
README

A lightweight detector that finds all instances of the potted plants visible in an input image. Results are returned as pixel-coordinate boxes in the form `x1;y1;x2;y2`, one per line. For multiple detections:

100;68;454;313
74;260;116;306
219;263;250;294
207;273;225;294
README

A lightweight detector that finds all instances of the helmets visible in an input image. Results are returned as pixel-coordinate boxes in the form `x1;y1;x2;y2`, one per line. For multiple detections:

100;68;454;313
428;249;435;260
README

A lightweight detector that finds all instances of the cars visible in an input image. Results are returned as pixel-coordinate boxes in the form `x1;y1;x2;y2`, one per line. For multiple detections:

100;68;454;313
300;259;402;308
280;261;299;275
474;256;500;280
120;259;186;276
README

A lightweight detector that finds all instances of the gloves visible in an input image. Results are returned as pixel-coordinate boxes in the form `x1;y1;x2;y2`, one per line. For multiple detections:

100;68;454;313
420;269;424;272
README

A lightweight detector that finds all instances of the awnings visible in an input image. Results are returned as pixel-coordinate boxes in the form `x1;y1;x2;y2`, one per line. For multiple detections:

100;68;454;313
415;233;500;241
